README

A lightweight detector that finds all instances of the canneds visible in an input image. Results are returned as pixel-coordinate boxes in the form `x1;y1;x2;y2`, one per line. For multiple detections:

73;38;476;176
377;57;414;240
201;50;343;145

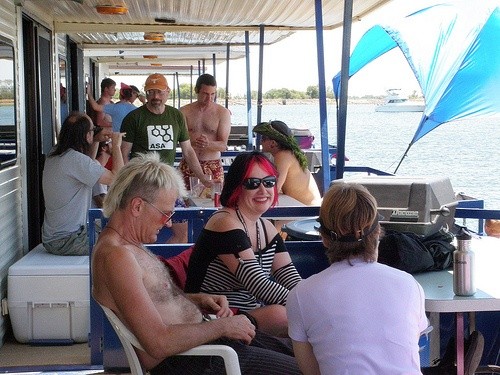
214;192;221;207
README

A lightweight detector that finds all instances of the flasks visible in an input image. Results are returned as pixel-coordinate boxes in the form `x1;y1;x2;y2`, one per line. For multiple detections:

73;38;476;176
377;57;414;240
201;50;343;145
452;227;477;297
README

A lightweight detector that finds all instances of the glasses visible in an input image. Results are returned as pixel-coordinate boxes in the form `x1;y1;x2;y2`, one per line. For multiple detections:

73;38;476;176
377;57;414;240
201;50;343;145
146;89;166;95
140;196;176;225
242;175;277;190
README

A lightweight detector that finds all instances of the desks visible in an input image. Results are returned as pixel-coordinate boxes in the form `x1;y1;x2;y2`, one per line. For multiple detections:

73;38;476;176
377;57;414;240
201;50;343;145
415;271;500;375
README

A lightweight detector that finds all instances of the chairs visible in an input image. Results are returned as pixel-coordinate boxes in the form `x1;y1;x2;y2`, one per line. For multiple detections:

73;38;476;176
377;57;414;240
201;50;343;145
96;302;243;375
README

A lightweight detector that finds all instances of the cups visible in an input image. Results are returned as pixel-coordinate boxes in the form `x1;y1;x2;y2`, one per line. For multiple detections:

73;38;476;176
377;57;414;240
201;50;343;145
202;174;212;181
189;177;201;197
211;181;223;201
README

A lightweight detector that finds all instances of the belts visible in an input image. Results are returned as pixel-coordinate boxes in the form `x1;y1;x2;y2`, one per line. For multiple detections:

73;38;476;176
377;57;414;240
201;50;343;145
202;311;212;323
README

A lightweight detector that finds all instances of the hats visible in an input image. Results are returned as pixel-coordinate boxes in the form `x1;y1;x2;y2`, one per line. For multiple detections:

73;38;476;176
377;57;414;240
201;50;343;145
144;73;168;92
252;121;309;171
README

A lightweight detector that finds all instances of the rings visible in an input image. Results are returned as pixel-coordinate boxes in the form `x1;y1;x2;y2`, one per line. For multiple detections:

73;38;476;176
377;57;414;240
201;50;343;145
202;145;204;148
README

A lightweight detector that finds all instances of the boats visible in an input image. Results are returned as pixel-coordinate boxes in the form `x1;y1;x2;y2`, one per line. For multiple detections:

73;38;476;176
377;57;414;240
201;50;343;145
375;89;425;112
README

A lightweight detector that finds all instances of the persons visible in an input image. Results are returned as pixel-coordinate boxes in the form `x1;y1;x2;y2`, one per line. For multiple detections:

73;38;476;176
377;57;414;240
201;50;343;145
286;183;429;375
60;83;66;124
42;112;126;256
86;73;322;256
91;151;303;375
186;153;302;337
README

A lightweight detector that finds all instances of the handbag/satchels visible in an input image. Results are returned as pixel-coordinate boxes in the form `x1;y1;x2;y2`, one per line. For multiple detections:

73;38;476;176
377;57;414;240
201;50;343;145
379;229;457;274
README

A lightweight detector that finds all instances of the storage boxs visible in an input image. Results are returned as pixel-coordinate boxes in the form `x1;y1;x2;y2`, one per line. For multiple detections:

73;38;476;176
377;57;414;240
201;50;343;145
329;178;456;238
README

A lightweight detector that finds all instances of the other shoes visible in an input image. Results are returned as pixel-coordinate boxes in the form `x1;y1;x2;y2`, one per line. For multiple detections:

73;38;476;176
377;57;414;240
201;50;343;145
433;329;484;375
